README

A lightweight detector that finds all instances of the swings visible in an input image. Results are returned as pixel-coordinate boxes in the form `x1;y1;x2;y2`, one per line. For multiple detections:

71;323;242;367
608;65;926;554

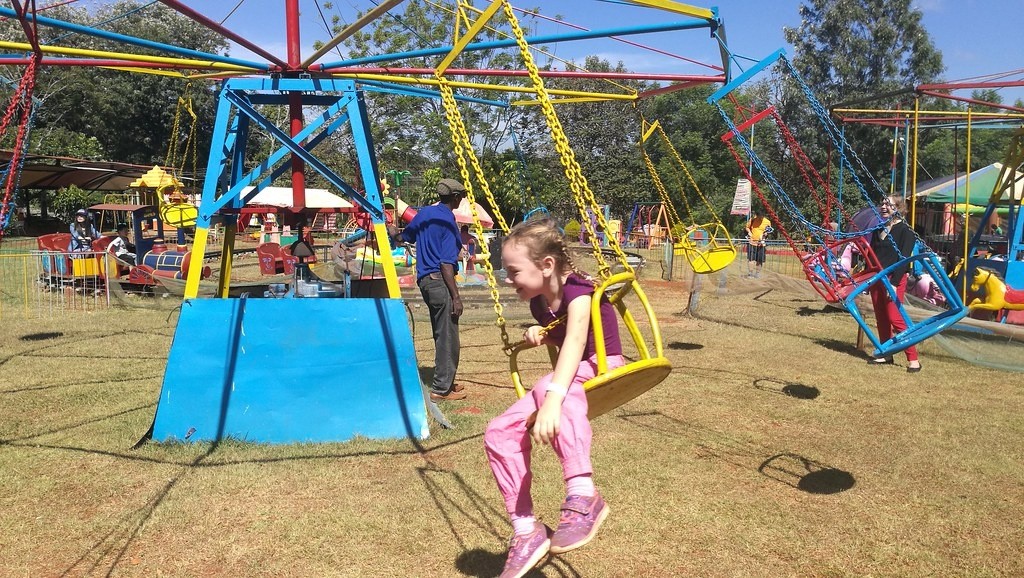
634;117;738;274
717;55;970;359
725;110;882;303
435;2;673;421
2;59;39;236
160;102;199;230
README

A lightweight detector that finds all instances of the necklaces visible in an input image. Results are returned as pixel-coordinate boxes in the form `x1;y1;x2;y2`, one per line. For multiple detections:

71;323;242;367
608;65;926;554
544;296;566;327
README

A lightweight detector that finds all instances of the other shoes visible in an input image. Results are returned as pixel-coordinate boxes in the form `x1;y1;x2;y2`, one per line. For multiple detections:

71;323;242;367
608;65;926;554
756;275;761;280
868;356;894;365
452;383;464;392
908;359;921;372
430;391;467;402
748;273;754;279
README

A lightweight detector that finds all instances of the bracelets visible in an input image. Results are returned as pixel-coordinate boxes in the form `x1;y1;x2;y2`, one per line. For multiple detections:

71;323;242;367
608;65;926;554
545;383;569;405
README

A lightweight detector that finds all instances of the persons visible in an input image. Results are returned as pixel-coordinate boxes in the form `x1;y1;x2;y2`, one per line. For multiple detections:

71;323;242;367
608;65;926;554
483;219;626;578
106;223;137;272
387;180;467;400
991;224;1002;236
745;208;771;279
461;225;481;254
864;194;922;373
68;209;102;261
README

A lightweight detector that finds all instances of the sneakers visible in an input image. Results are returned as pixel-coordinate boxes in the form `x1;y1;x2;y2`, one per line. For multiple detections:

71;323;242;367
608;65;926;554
551;491;610;554
498;516;555;578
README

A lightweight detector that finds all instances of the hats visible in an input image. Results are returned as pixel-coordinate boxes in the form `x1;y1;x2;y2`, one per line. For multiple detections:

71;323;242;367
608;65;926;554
438;179;470;196
76;209;88;216
